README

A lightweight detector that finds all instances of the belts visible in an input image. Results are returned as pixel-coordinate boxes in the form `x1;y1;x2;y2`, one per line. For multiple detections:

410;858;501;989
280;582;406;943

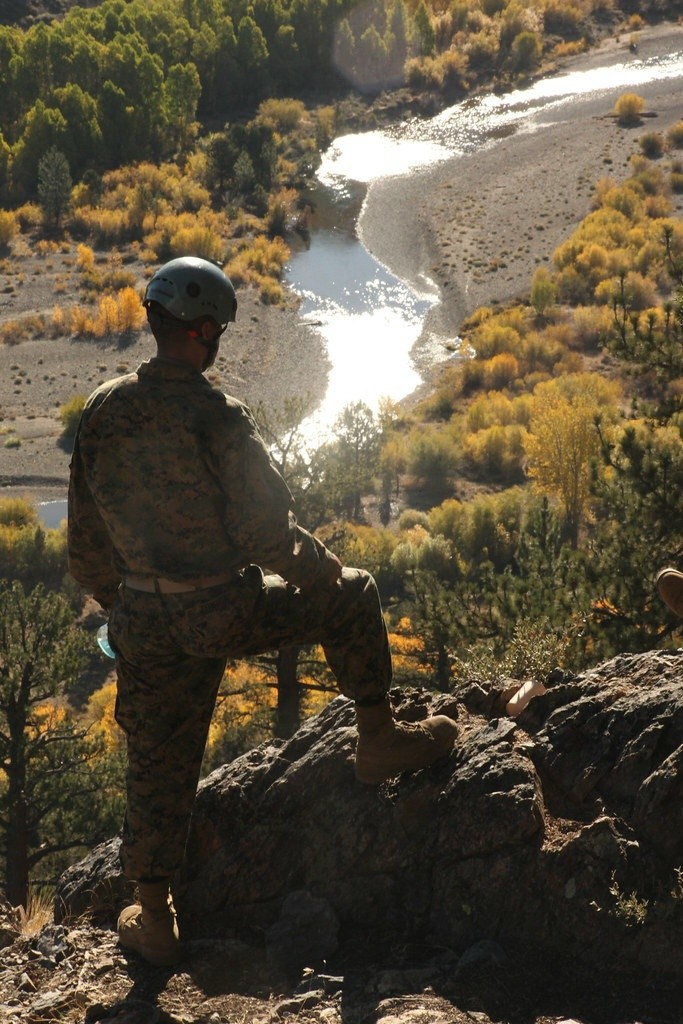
122;572;239;596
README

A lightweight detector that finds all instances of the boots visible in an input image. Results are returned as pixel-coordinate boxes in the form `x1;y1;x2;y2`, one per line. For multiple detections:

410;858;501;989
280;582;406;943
117;877;176;960
355;697;458;780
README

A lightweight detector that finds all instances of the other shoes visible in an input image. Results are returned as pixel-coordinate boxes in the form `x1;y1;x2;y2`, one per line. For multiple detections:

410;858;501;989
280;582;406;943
657;568;683;618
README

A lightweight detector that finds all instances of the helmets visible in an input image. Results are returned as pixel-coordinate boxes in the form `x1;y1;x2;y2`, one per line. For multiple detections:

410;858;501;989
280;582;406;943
142;257;237;324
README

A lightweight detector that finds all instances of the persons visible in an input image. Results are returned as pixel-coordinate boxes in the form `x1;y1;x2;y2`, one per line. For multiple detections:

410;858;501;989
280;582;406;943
656;566;682;620
62;253;459;971
